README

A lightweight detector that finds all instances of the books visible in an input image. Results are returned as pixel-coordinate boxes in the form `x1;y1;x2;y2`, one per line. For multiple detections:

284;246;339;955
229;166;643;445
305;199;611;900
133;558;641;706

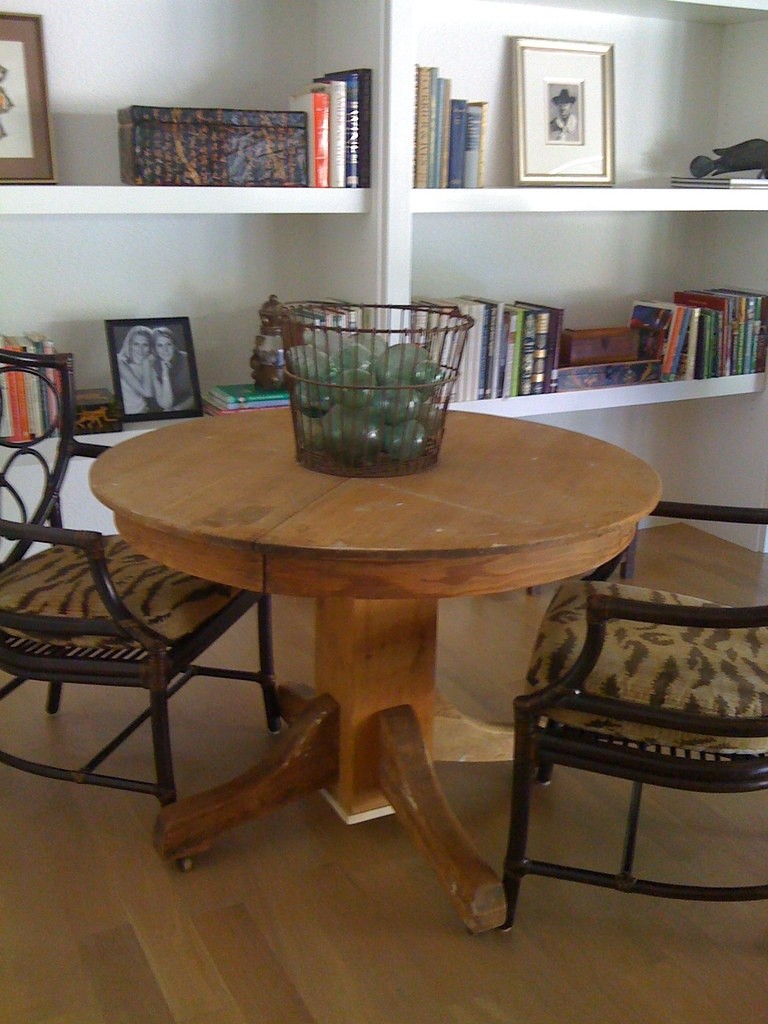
414;65;490;188
409;285;768;404
200;298;360;416
669;175;768;189
0;331;66;440
288;67;372;188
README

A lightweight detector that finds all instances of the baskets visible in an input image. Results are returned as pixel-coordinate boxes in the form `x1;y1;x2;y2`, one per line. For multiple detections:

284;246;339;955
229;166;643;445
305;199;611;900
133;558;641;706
279;301;475;478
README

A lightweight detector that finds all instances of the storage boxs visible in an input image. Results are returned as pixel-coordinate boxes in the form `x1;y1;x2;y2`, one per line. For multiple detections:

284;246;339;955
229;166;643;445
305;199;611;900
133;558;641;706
74;388;122;435
117;105;310;187
558;326;661;393
557;326;661;392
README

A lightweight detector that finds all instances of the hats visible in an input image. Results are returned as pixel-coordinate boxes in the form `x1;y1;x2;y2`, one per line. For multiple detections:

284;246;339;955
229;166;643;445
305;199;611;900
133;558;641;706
553;89;576;107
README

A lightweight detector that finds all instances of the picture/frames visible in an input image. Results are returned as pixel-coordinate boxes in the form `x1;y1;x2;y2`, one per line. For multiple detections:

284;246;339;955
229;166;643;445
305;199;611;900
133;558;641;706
509;36;616;187
0;12;58;184
105;316;203;421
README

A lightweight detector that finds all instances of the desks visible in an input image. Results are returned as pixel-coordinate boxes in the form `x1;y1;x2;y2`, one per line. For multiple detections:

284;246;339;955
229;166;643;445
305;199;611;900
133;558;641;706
89;406;663;936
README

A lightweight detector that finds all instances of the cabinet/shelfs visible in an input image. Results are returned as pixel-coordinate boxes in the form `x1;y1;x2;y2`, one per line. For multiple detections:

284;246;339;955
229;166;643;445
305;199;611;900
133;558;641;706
0;0;768;561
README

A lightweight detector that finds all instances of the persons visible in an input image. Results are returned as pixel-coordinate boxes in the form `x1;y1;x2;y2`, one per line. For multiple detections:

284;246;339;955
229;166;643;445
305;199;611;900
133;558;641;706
115;326;194;415
548;87;584;144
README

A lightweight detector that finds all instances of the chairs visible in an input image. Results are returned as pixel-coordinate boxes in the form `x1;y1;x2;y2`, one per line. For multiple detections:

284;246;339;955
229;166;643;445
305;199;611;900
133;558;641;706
499;501;768;931
0;348;281;807
